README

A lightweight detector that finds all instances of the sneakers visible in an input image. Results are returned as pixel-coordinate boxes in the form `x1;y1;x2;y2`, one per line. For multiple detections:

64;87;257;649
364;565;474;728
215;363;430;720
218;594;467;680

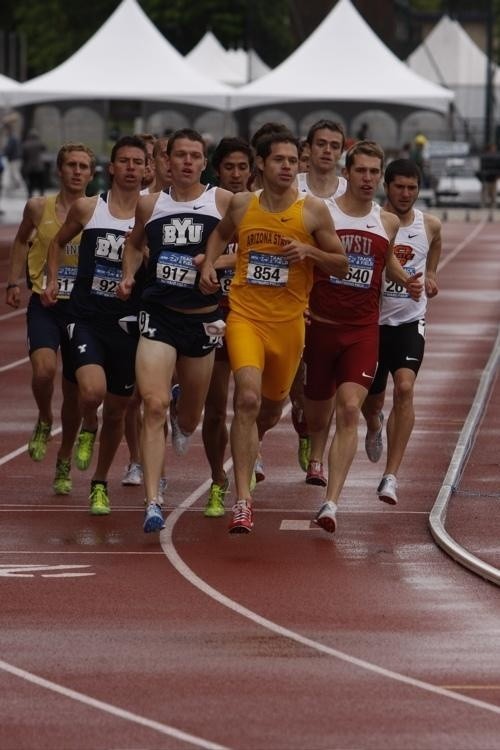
121;463;143;486
365;412;384;462
170;400;187;452
312;501;338;533
249;459;257;493
306;460;328;487
298;436;311;472
53;456;72;495
28;417;52;461
376;473;396;505
87;484;110;515
142;503;167;533
172;384;195;436
73;415;98;470
144;477;168;506
255;441;265;483
204;469;229;518
228;499;255;533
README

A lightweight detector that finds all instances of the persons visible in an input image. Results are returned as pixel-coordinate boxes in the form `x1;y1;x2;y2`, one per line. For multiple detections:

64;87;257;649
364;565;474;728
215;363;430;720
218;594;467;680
0;121;498;535
